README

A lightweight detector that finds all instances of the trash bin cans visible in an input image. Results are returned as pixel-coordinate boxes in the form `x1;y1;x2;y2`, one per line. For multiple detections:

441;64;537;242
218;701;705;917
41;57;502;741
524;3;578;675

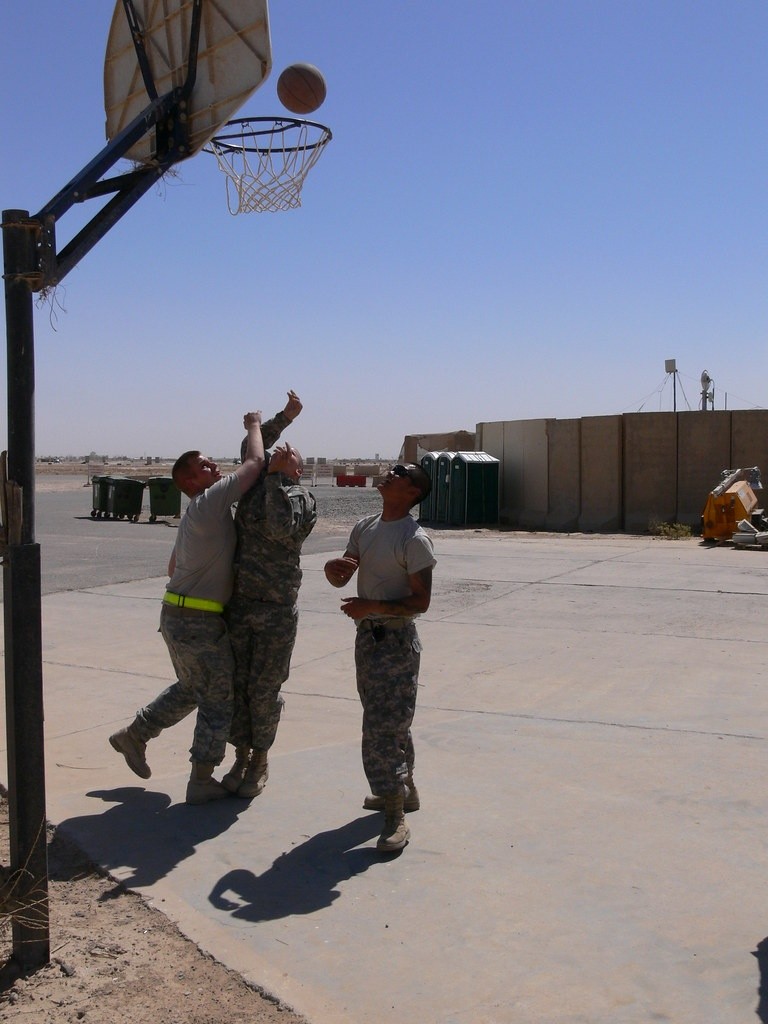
104;477;146;522
90;474;113;518
146;476;181;522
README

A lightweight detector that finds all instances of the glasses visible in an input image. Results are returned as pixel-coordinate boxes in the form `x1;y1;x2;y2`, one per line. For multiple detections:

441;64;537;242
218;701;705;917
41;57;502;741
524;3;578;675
392;464;419;488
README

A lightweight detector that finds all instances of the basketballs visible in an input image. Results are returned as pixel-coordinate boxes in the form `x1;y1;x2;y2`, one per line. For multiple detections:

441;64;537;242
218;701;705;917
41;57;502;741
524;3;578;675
277;63;327;115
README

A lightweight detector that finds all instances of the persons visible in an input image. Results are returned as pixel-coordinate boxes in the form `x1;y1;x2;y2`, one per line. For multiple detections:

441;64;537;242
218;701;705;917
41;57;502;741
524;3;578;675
222;388;317;796
109;409;265;804
324;459;437;851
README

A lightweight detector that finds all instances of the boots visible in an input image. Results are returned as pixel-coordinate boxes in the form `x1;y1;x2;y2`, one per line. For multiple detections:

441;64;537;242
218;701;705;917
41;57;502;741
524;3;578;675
238;745;268;799
185;755;231;805
376;796;411;853
221;742;255;794
363;775;420;811
108;718;152;779
